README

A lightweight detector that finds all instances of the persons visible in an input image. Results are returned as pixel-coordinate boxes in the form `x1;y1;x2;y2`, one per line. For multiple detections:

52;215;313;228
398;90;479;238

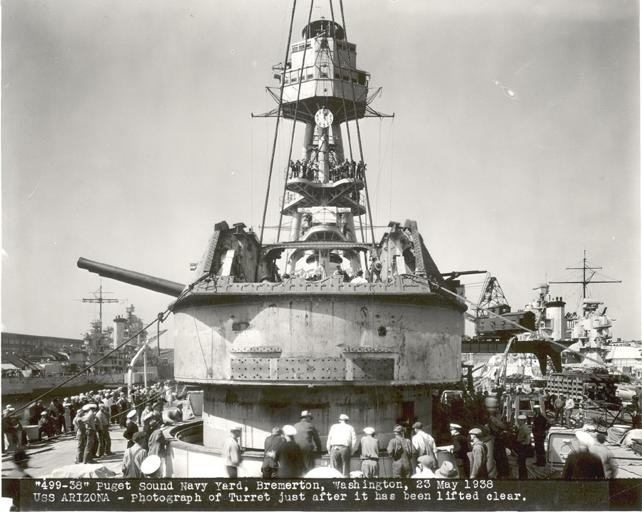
350;270;368;283
333;264;351;282
2;380;641;480
289;160;367;182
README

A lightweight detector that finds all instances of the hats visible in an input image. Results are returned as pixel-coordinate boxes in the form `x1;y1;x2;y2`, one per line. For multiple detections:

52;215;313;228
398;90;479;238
449;423;462;430
13;450;29;463
364;427;376;434
417;455;434;466
64;387;154;422
282;425;297;435
338;413;352;421
393;426;402;432
5;404;15;411
40;411;47;416
300;410;313;419
412;422;422;429
469;428;482;434
132;432;146;442
435;461;459;478
140;455;161;474
